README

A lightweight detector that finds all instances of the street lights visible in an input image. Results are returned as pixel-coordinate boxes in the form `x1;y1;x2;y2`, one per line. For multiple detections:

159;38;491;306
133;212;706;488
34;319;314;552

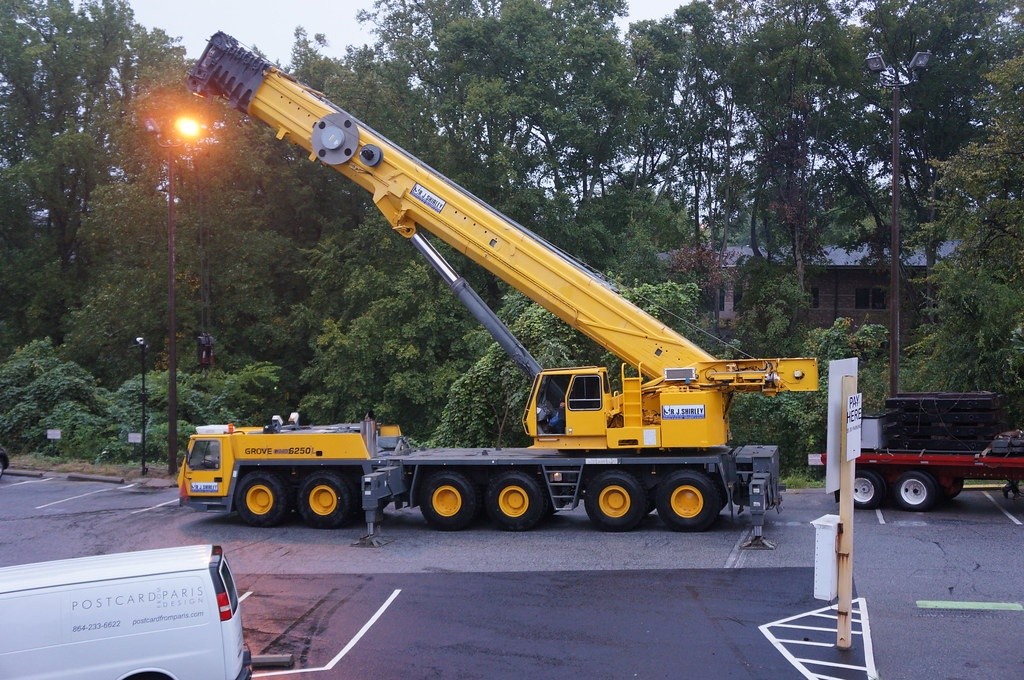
144;118;202;475
866;52;933;399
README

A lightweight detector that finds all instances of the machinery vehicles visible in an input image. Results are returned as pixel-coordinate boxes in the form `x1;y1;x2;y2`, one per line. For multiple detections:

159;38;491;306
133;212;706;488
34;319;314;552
178;29;818;549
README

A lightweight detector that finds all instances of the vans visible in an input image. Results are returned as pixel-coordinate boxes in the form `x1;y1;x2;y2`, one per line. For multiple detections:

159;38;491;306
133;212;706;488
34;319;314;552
0;544;253;680
